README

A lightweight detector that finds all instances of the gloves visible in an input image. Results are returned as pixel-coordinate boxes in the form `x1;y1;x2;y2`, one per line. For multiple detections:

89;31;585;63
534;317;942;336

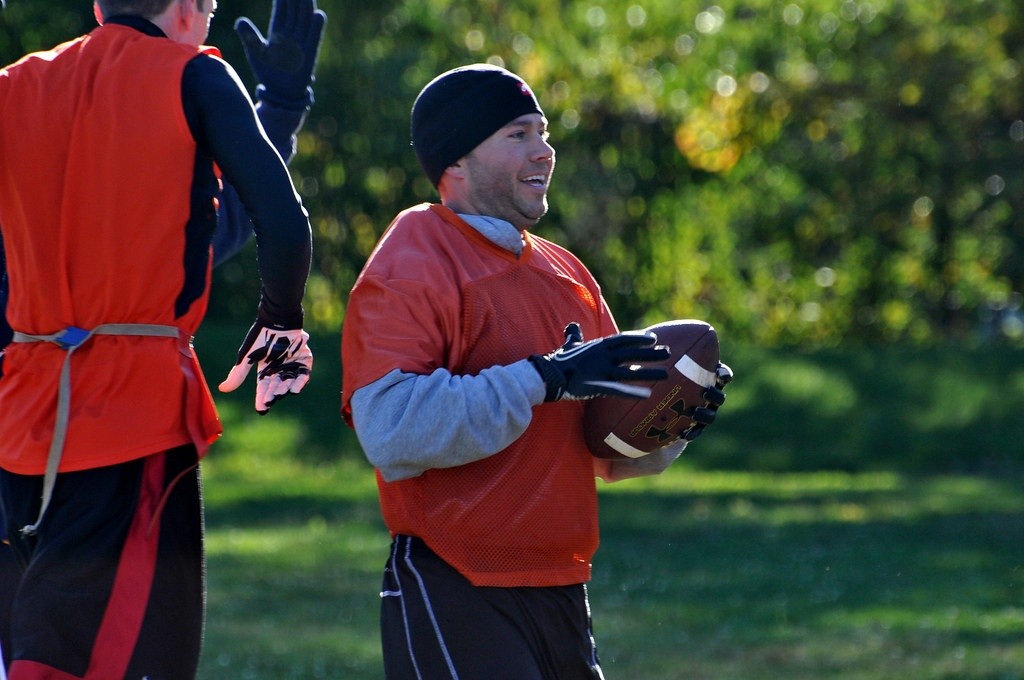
217;317;314;416
526;321;673;404
681;360;734;441
234;0;328;106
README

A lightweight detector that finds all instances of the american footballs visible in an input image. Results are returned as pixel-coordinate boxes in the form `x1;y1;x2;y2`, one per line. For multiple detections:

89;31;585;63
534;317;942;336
582;319;719;461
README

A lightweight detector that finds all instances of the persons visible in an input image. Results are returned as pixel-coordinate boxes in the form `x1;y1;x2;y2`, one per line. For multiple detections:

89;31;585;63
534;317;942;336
339;65;733;680
0;0;329;680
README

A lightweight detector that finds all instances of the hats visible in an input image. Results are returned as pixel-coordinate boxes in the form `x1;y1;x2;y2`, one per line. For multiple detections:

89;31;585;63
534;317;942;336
410;63;545;192
